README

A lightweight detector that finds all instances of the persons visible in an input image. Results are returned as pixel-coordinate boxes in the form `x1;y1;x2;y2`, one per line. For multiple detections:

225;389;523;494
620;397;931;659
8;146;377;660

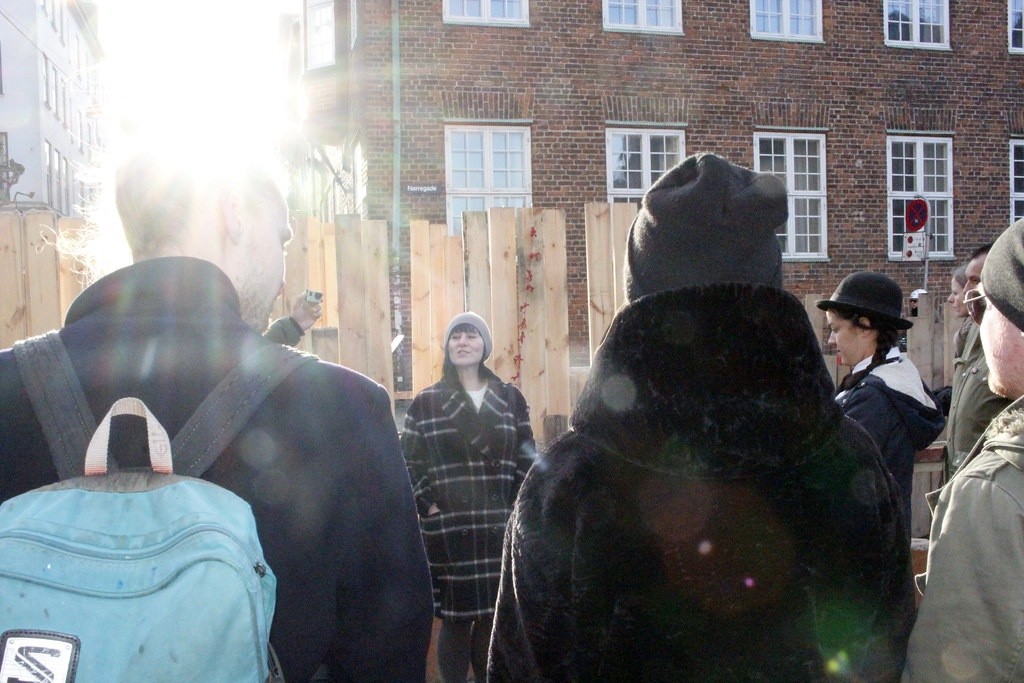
817;273;947;496
400;312;536;683
901;218;1023;683
487;156;916;683
0;149;435;683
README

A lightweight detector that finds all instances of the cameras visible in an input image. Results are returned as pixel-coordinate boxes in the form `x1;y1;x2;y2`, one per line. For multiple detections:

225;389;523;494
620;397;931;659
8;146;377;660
305;290;322;303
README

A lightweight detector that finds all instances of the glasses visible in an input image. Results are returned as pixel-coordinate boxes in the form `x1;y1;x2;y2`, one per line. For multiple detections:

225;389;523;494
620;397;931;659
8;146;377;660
910;299;917;303
961;290;987;326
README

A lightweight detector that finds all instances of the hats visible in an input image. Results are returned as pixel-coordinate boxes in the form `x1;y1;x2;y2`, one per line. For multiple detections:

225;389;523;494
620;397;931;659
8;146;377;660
622;154;790;309
911;289;928;297
442;311;493;363
980;216;1023;332
815;271;913;334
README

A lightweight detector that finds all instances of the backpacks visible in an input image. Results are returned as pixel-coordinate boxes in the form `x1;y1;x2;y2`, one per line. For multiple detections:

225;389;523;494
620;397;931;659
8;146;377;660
0;331;320;682
